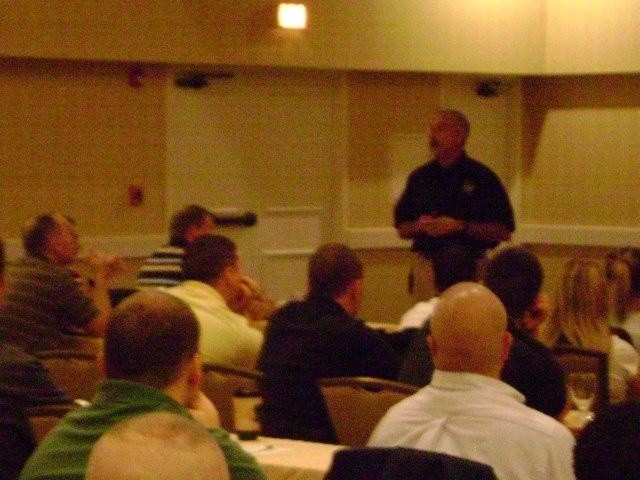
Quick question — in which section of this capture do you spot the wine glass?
[566,374,602,413]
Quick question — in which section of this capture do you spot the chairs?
[1,322,640,478]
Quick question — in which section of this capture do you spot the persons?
[0,205,638,450]
[0,240,75,478]
[366,280,579,479]
[394,112,515,294]
[81,408,231,479]
[571,364,639,480]
[18,289,266,479]
